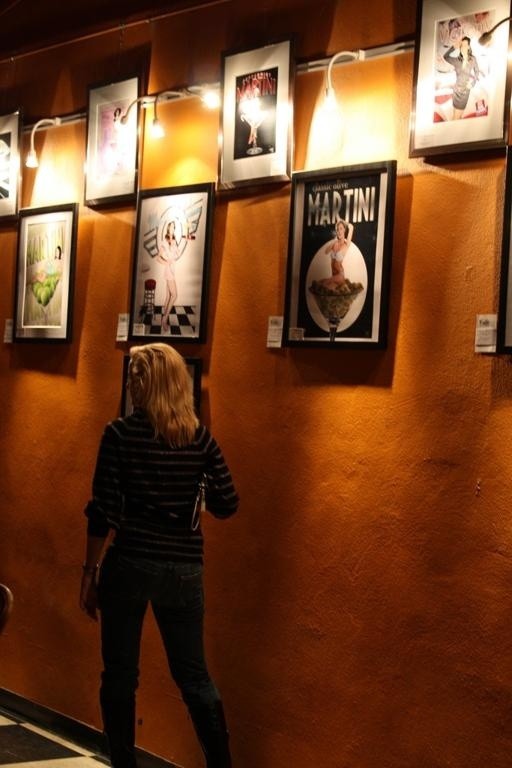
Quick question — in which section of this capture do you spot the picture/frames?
[127,181,216,344]
[0,104,21,229]
[405,0,512,160]
[81,67,144,208]
[213,29,300,198]
[494,145,512,356]
[12,202,78,344]
[281,160,399,349]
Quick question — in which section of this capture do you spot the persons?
[443,36,479,121]
[110,107,123,140]
[245,79,259,148]
[157,221,189,330]
[324,220,355,293]
[48,244,63,278]
[78,341,241,766]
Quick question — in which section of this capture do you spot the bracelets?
[82,561,100,572]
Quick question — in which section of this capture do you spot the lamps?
[316,44,366,106]
[23,115,61,168]
[114,80,220,139]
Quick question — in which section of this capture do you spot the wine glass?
[305,286,363,342]
